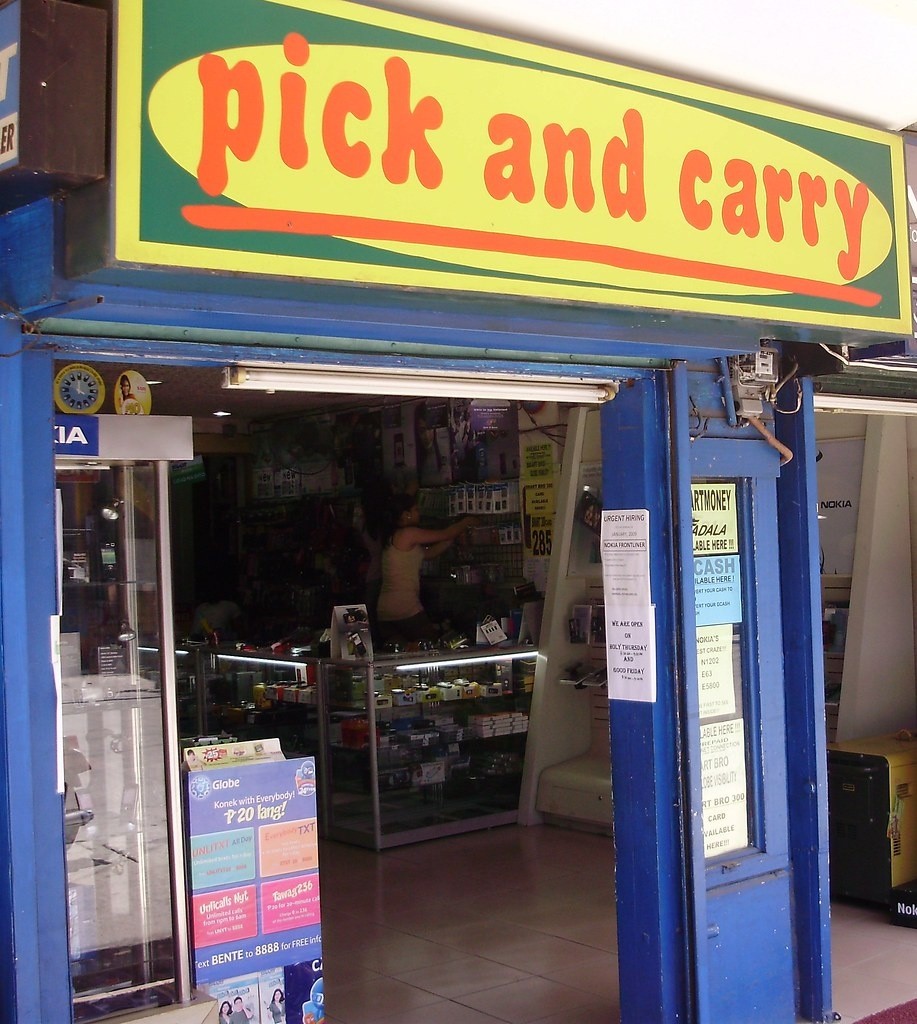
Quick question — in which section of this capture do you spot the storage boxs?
[327,666,529,793]
[230,672,316,703]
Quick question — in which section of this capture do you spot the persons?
[119,375,135,406]
[374,494,480,642]
[219,996,253,1024]
[191,585,243,641]
[186,750,207,766]
[264,989,286,1024]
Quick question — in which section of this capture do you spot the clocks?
[52,362,105,414]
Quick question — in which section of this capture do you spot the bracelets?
[267,1007,270,1010]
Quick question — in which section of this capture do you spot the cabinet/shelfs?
[200,641,330,842]
[51,414,220,1024]
[321,644,540,851]
[135,636,204,739]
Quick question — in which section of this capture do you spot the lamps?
[219,366,620,405]
[116,619,135,641]
[101,497,120,521]
